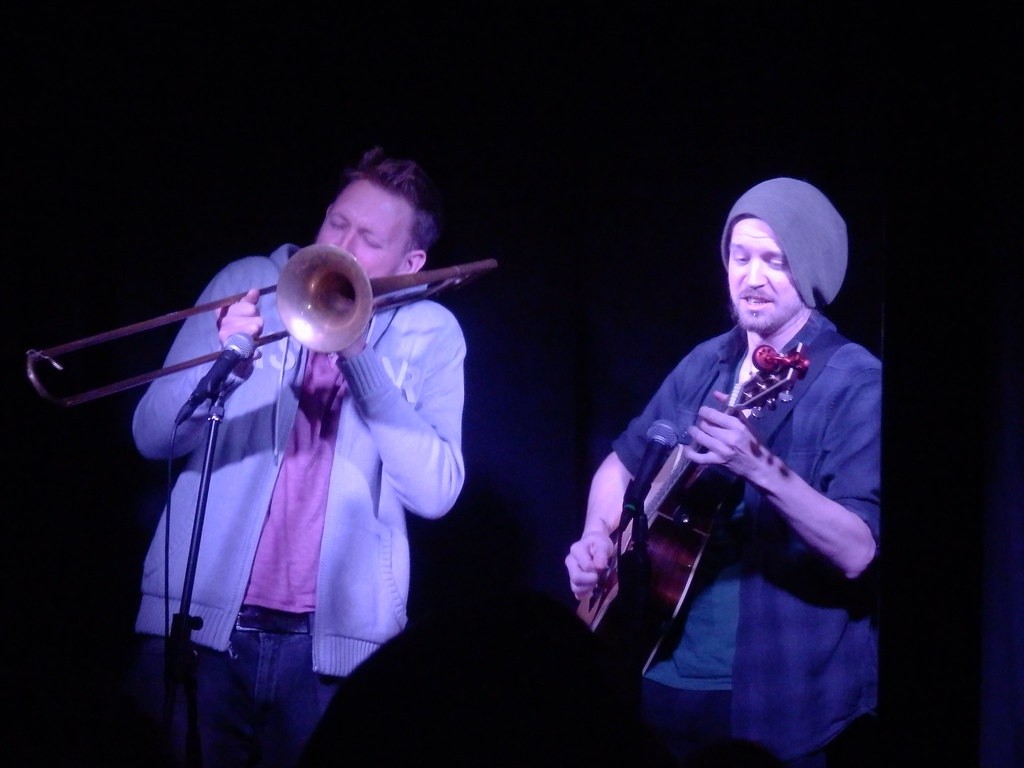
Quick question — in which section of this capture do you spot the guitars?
[575,342,812,677]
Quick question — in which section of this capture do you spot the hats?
[721,177,850,308]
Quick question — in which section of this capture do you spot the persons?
[565,176,884,768]
[128,153,467,768]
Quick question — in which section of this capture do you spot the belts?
[234,604,311,634]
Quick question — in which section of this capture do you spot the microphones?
[173,331,257,424]
[620,420,679,532]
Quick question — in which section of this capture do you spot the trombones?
[26,242,500,412]
